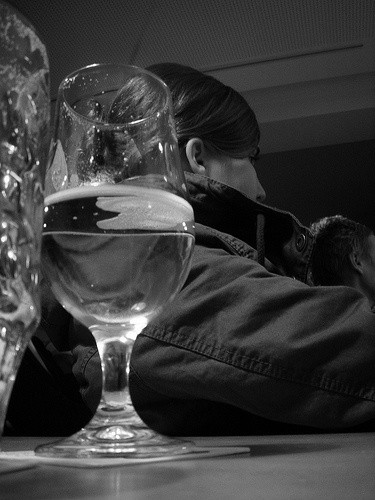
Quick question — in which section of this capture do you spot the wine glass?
[33,62,195,459]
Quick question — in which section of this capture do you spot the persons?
[0,63,375,436]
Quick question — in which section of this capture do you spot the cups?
[0,2,51,435]
